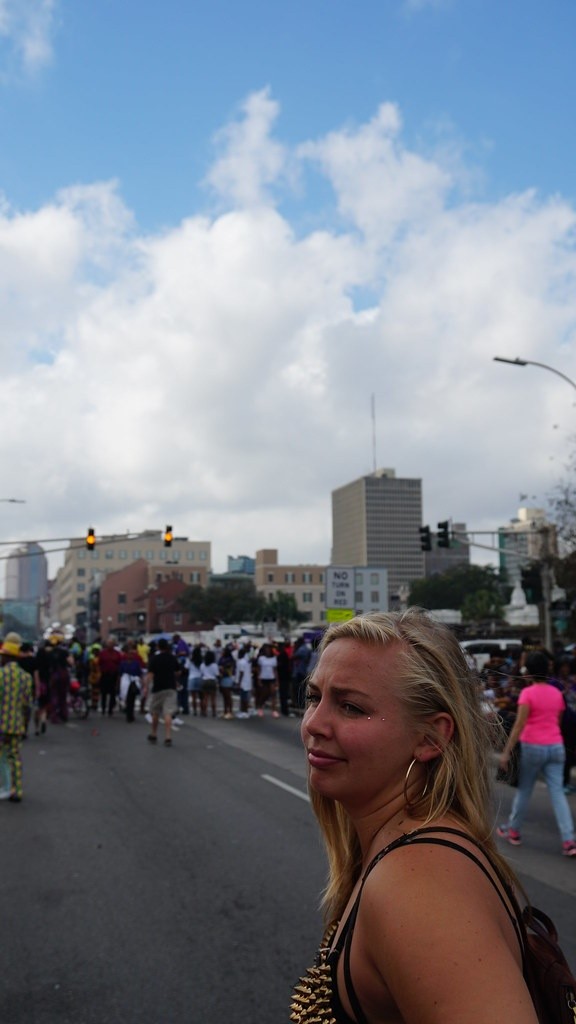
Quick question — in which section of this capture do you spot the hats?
[1,641,20,658]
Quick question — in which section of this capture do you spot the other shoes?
[147,734,157,744]
[235,711,249,720]
[172,718,183,730]
[250,709,256,716]
[272,710,280,718]
[218,711,231,721]
[258,711,262,714]
[144,713,164,724]
[164,739,173,747]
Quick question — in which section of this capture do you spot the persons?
[288,607,539,1024]
[0,624,576,857]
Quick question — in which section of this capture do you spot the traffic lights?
[86,527,95,550]
[164,526,174,546]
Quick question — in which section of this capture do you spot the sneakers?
[560,840,576,856]
[497,826,522,846]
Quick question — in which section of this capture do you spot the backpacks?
[521,899,576,1024]
